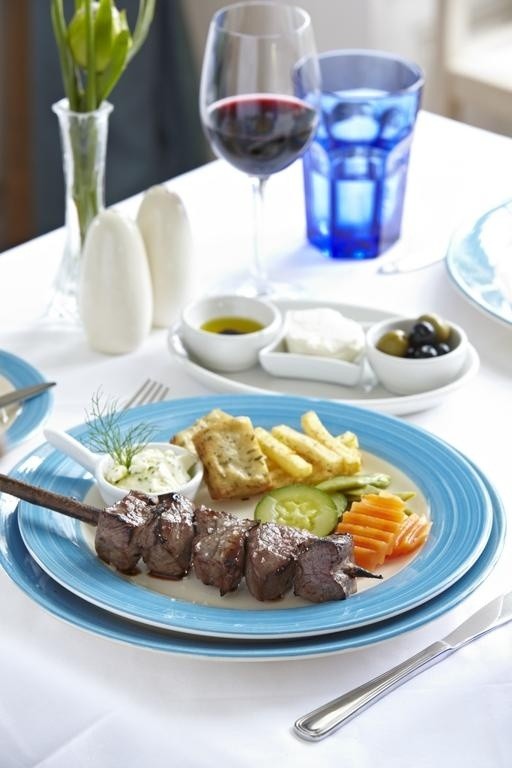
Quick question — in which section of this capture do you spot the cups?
[168,292,282,374]
[289,48,424,261]
[365,313,471,395]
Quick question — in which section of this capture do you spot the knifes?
[288,590,511,744]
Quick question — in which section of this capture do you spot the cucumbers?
[254,482,336,537]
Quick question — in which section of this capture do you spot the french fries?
[255,409,361,480]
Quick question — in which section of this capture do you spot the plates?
[0,394,507,663]
[0,349,53,456]
[444,193,512,330]
[172,304,479,417]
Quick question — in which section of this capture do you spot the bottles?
[76,185,204,355]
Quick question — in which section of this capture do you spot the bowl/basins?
[41,424,205,509]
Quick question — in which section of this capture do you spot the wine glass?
[198,3,322,304]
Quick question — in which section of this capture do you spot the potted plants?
[53,0,158,293]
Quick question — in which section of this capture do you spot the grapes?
[405,322,448,356]
